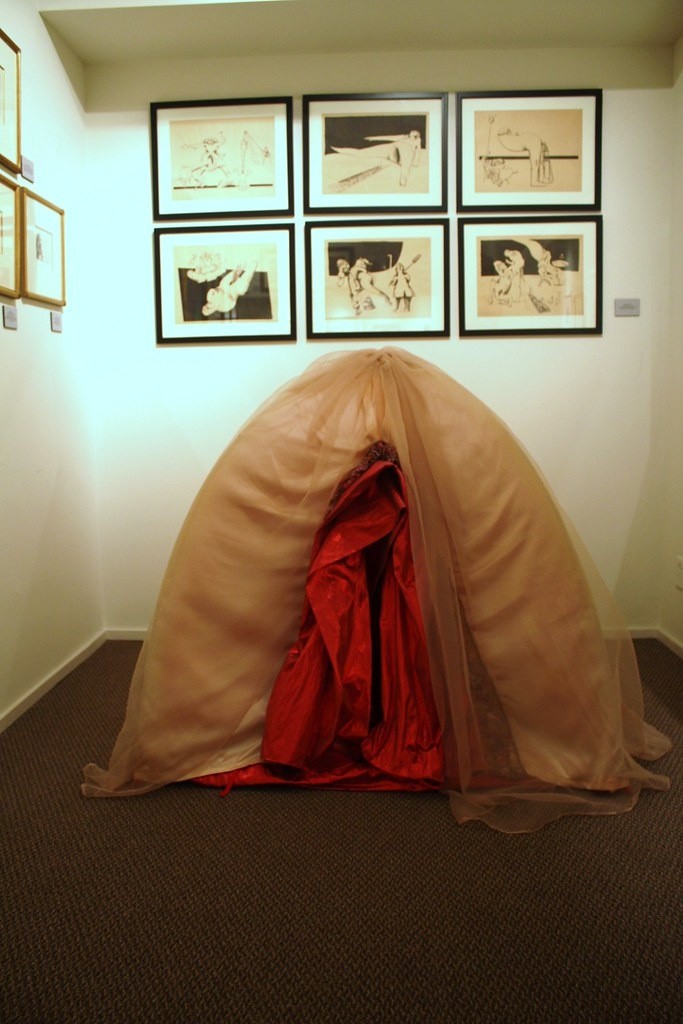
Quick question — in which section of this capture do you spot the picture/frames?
[305,221,450,339]
[150,97,295,220]
[304,94,449,212]
[457,217,603,336]
[0,175,20,300]
[21,185,66,306]
[456,90,602,211]
[153,224,296,344]
[0,27,23,173]
